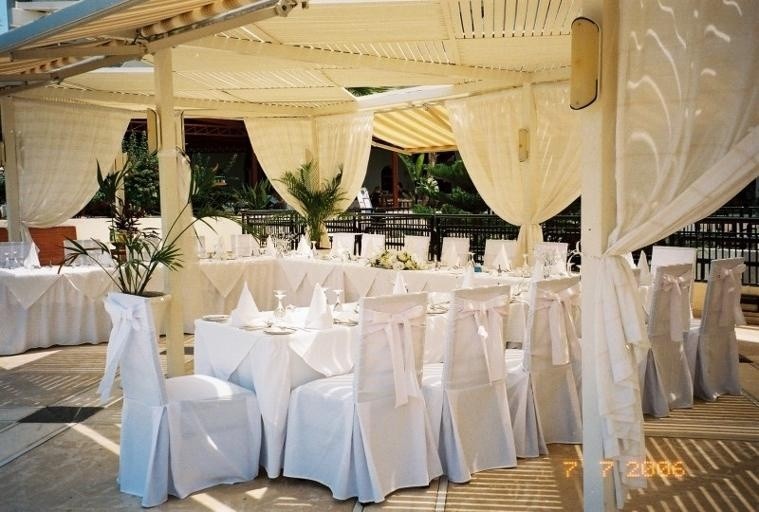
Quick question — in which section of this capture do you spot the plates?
[243,321,270,330]
[266,327,294,334]
[423,305,449,314]
[203,313,230,322]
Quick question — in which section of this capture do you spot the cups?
[322,288,328,305]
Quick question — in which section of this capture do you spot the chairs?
[1,233,582,364]
[421,283,517,485]
[504,275,582,459]
[650,245,697,281]
[641,262,695,418]
[687,256,744,403]
[95,292,263,507]
[283,290,444,505]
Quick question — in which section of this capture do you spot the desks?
[191,292,530,479]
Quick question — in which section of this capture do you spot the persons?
[369,186,387,208]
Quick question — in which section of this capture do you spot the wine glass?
[332,289,345,316]
[460,251,531,278]
[3,251,21,270]
[268,237,359,264]
[273,289,288,317]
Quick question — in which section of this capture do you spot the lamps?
[569,16,602,110]
[176,110,186,154]
[518,128,528,162]
[147,107,161,156]
